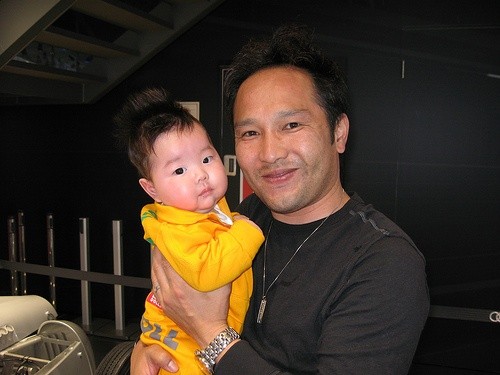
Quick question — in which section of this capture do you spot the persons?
[129,22,431,375]
[112,89,266,375]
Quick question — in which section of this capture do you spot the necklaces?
[256,188,345,324]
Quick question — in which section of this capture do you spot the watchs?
[194,327,241,375]
